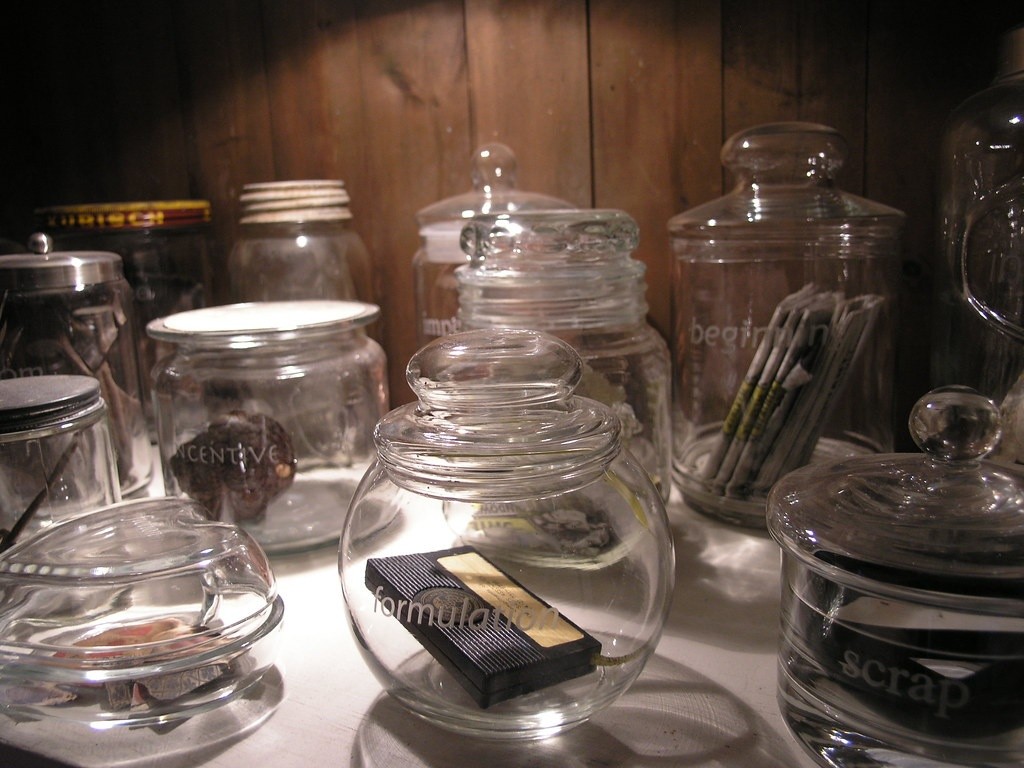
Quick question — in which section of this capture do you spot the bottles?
[0,26,1024,767]
